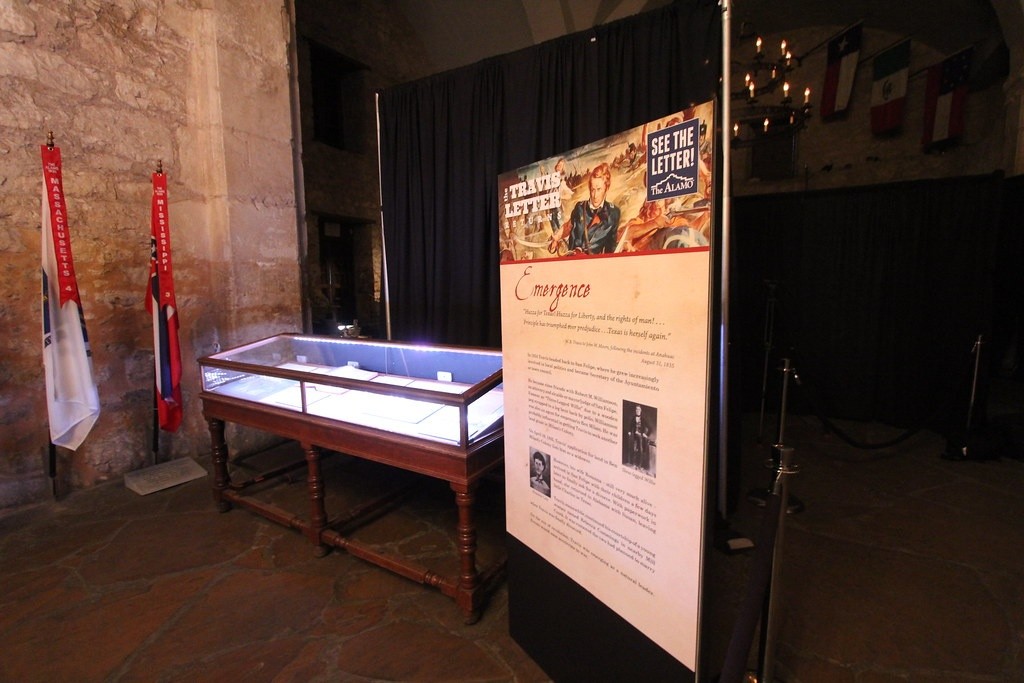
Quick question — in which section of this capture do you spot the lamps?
[719,13,813,148]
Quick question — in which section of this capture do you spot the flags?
[39,143,102,451]
[145,172,183,431]
